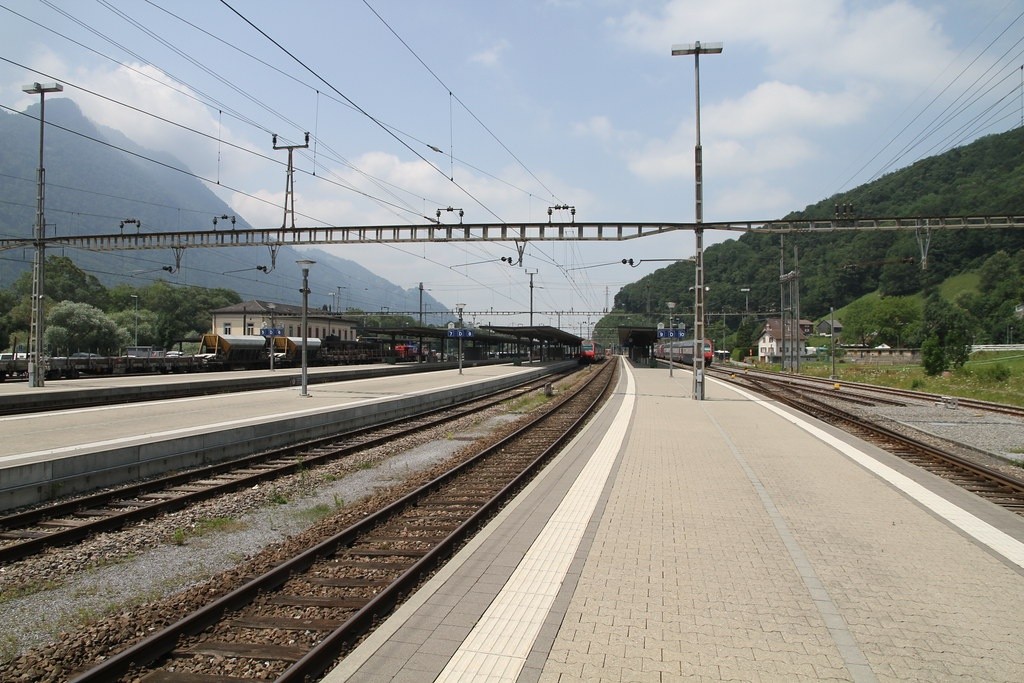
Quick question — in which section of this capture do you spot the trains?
[0,335,437,384]
[648,339,713,366]
[580,340,605,363]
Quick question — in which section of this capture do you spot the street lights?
[667,302,676,376]
[131,294,138,357]
[296,260,317,396]
[456,303,467,375]
[22,82,62,387]
[266,302,276,369]
[671,41,725,399]
